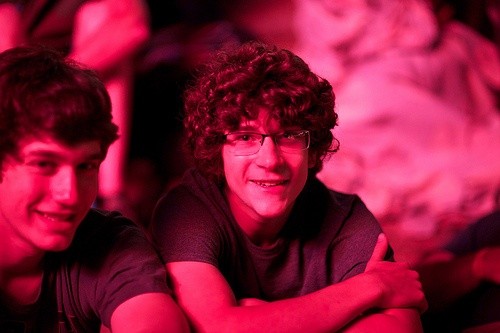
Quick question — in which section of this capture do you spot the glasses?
[216,129,310,156]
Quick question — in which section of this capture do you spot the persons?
[411,205,500,333]
[0,0,154,246]
[1,45,191,332]
[150,37,429,331]
